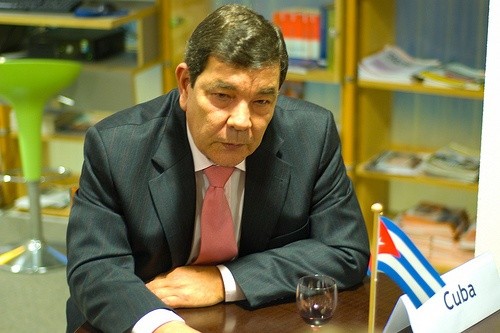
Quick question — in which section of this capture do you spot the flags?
[367,217,445,308]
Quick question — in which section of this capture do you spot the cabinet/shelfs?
[0,1,163,215]
[165,0,492,274]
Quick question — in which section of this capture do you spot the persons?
[65,5,370,333]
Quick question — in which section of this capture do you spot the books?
[364,152,479,181]
[359,47,485,90]
[391,201,476,252]
[272,9,335,69]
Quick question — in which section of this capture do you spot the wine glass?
[297,273,337,333]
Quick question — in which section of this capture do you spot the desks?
[72,271,500,333]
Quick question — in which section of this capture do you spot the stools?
[0,60,83,274]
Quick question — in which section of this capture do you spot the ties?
[193,166,237,266]
